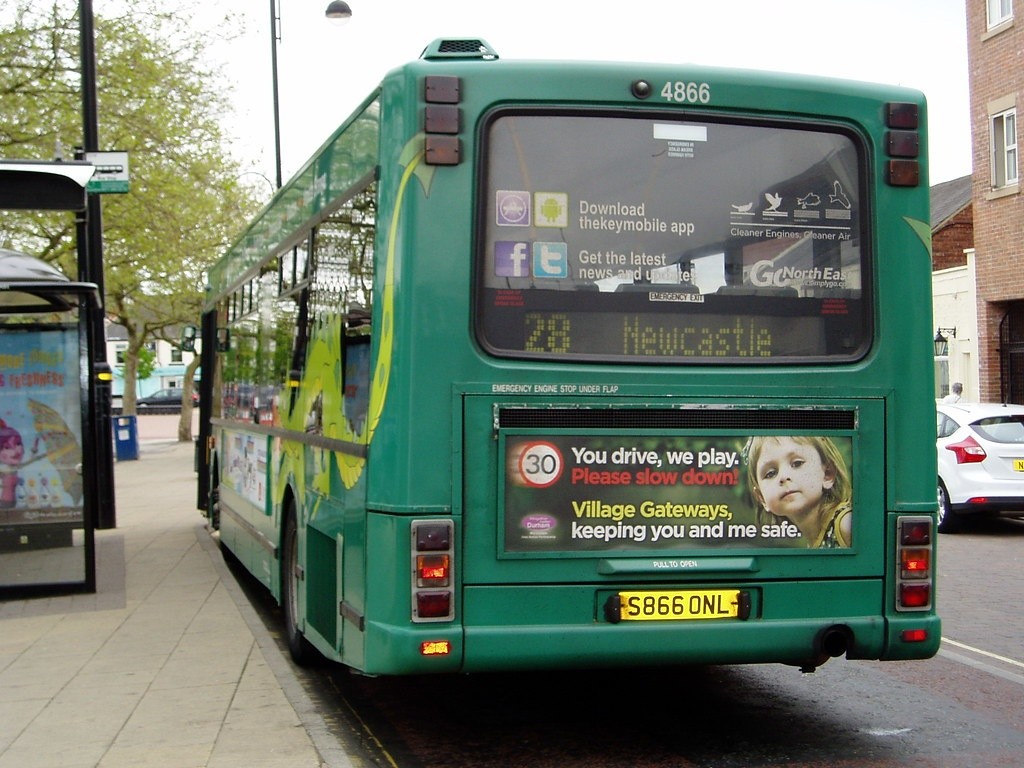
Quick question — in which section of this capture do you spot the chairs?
[615,284,700,293]
[813,288,861,300]
[716,286,799,297]
[487,280,600,292]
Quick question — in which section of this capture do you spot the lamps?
[934,326,956,356]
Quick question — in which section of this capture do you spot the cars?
[934,400,1024,534]
[136,387,201,416]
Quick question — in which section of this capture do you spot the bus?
[180,42,940,681]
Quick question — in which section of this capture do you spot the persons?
[938,382,962,437]
[747,436,852,549]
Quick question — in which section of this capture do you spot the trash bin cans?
[112,414,139,461]
